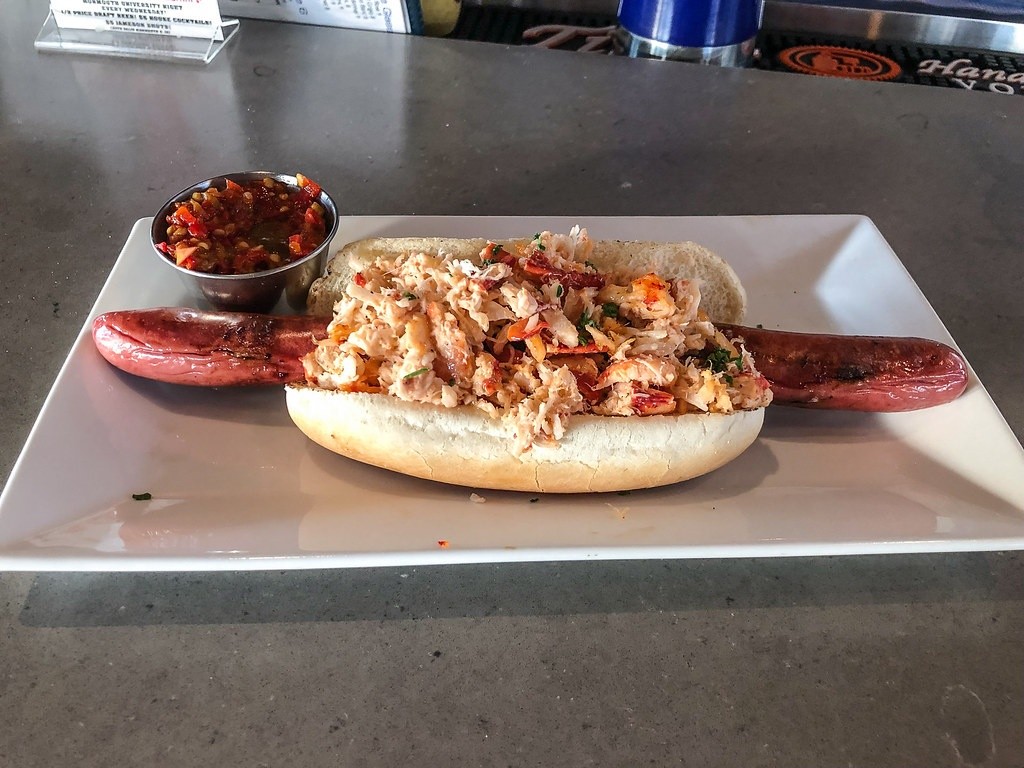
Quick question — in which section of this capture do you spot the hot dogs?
[86,225,968,496]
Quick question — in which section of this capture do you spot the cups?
[611,0,765,68]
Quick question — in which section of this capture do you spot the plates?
[0,215,1024,574]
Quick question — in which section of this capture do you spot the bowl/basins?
[152,171,339,316]
